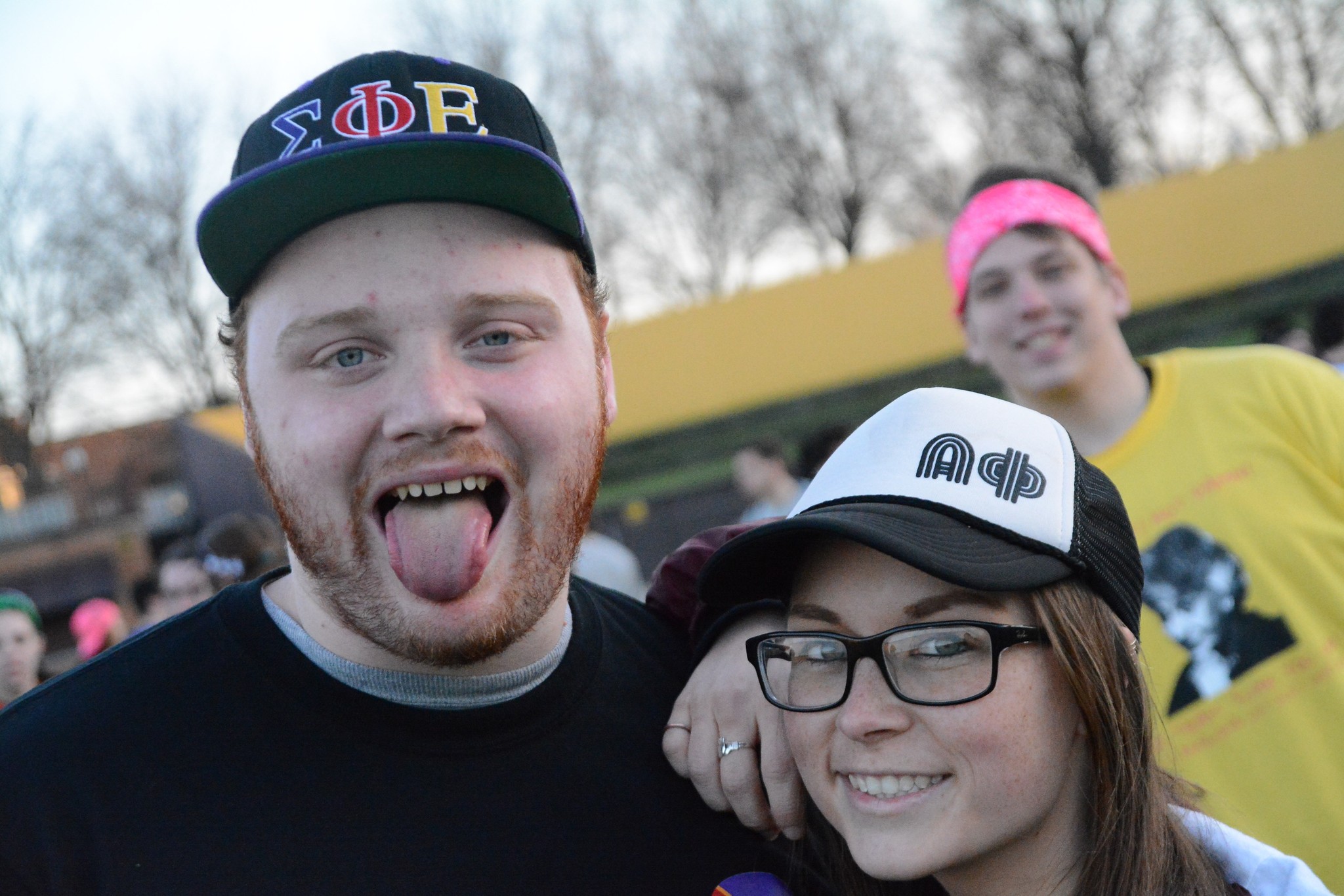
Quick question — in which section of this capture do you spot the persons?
[0,508,287,713]
[726,427,814,527]
[710,384,1330,896]
[943,159,1344,894]
[0,49,855,896]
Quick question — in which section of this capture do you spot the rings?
[718,737,758,757]
[665,722,692,735]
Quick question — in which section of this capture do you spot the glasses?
[746,618,1054,715]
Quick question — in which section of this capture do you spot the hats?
[693,387,1141,653]
[71,597,118,661]
[0,587,47,638]
[195,51,597,311]
